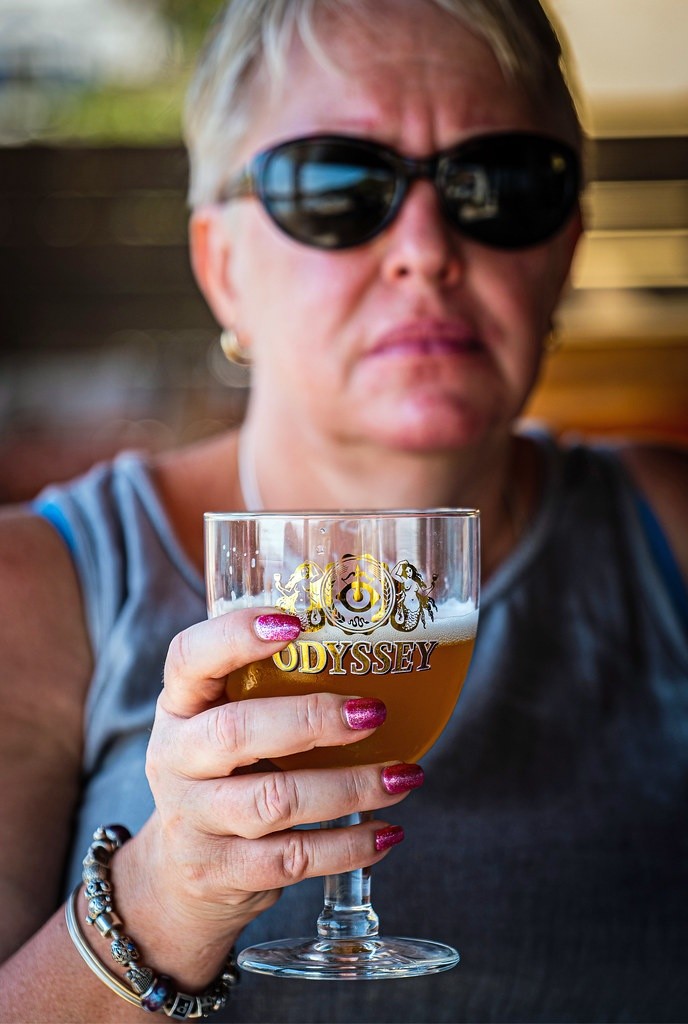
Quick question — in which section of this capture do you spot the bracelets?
[82,821,242,1017]
[61,884,151,1015]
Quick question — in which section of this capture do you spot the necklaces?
[235,424,522,611]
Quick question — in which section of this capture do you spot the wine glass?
[202,505,475,982]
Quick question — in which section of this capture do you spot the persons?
[0,0,688,1022]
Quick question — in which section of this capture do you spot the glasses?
[205,128,587,252]
